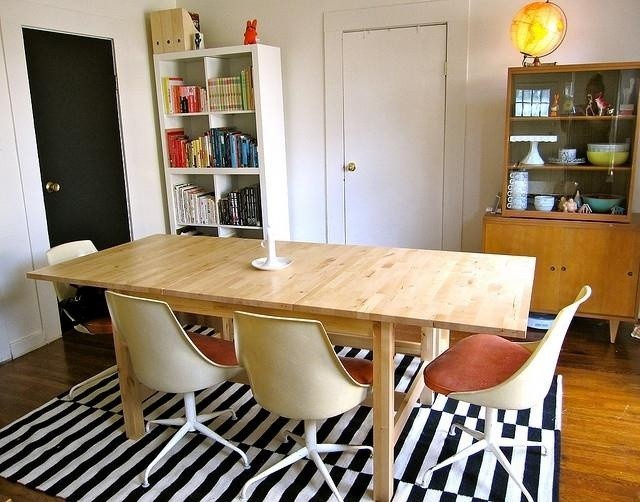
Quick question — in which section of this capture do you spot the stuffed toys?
[244,19,257,45]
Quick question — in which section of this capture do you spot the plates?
[548,158,585,165]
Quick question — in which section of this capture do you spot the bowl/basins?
[534,196,555,211]
[587,151,629,166]
[587,143,630,151]
[582,193,625,212]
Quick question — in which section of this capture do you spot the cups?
[557,149,577,160]
[507,171,529,210]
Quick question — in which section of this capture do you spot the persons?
[194,33,201,50]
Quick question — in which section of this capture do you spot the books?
[218,182,262,227]
[166,126,259,169]
[208,66,255,112]
[173,182,218,225]
[162,76,207,114]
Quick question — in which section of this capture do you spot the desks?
[27,234,538,440]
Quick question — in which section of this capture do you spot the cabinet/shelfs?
[484,211,640,344]
[150,42,290,239]
[500,59,638,222]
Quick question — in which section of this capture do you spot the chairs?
[232,311,373,502]
[46,238,113,338]
[420,285,593,501]
[105,289,251,489]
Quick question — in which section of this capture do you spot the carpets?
[2,321,565,501]
